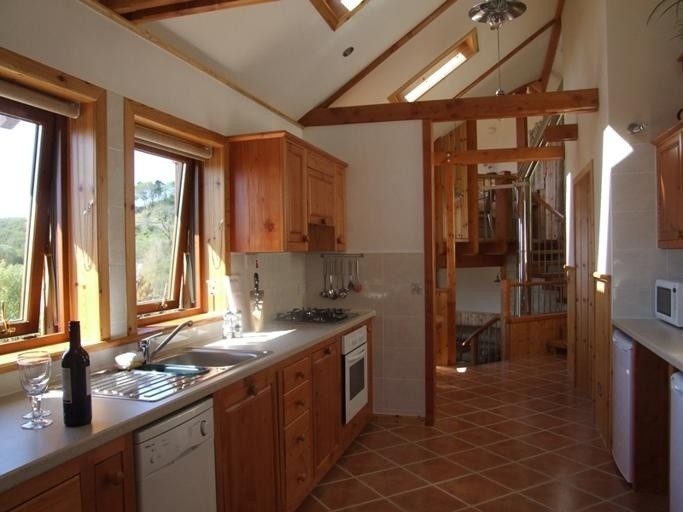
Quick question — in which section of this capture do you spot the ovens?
[342,325,369,425]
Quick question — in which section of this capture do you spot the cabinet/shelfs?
[310,143,335,227]
[230,131,309,256]
[309,155,350,252]
[343,405,370,448]
[0,432,136,511]
[277,349,317,511]
[611,313,683,509]
[313,333,343,488]
[592,273,610,450]
[214,362,277,511]
[650,123,683,247]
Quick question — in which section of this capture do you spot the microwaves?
[654,279,683,327]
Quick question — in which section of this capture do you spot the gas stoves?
[274,306,360,326]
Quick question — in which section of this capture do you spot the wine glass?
[19,359,52,429]
[18,351,50,418]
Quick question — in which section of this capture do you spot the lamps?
[468,1,527,95]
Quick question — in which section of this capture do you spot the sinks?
[88,363,209,380]
[156,348,258,368]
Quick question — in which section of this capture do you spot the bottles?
[232,307,242,336]
[222,309,233,338]
[61,321,92,427]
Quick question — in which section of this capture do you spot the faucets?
[140,321,195,360]
